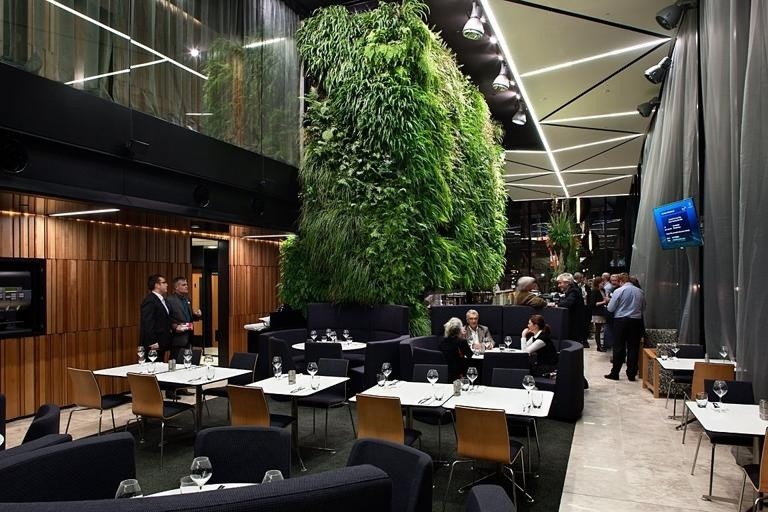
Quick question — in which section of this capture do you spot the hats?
[518,277,535,291]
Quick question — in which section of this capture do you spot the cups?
[273,367,282,380]
[474,348,480,356]
[661,349,669,361]
[310,376,322,391]
[179,476,202,493]
[434,387,446,402]
[262,469,285,485]
[460,378,469,390]
[529,392,545,411]
[695,391,708,409]
[375,372,386,387]
[347,337,353,345]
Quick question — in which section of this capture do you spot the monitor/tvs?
[652,196,704,250]
[0,257,46,339]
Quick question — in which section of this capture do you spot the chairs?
[20,402,62,444]
[196,426,293,484]
[413,363,457,450]
[352,342,400,386]
[346,437,435,510]
[356,395,435,490]
[492,368,542,473]
[638,329,768,512]
[482,351,529,387]
[64,367,146,447]
[198,352,259,421]
[174,349,212,418]
[303,342,343,373]
[268,335,297,377]
[297,359,357,446]
[441,406,527,510]
[127,373,197,471]
[467,484,519,510]
[226,384,296,465]
[413,347,445,367]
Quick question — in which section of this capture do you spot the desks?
[291,341,368,352]
[350,379,457,454]
[246,371,350,473]
[155,363,253,437]
[93,361,189,381]
[476,346,522,358]
[441,384,555,418]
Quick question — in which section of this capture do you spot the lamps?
[637,97,661,117]
[492,62,510,92]
[462,3,484,41]
[655,0,696,30]
[644,56,671,84]
[512,99,526,125]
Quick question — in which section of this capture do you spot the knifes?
[289,386,305,393]
[388,380,399,386]
[417,396,431,404]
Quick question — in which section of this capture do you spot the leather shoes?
[626,369,635,381]
[605,374,619,380]
[597,348,607,352]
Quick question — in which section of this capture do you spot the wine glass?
[428,368,438,394]
[466,367,478,392]
[272,356,283,382]
[381,362,391,382]
[307,362,319,380]
[670,341,680,361]
[308,327,349,345]
[711,379,729,409]
[719,345,729,363]
[482,335,513,351]
[522,375,538,401]
[114,478,143,499]
[183,348,215,380]
[189,456,214,491]
[136,345,159,373]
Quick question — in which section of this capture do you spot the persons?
[165,276,203,399]
[462,309,496,352]
[548,267,646,390]
[443,317,473,360]
[515,275,546,308]
[521,315,557,376]
[140,272,171,363]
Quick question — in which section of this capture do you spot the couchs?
[401,302,583,425]
[256,302,411,393]
[4,431,393,510]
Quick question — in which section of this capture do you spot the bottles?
[453,379,461,395]
[759,399,768,419]
[288,369,296,384]
[168,359,176,370]
